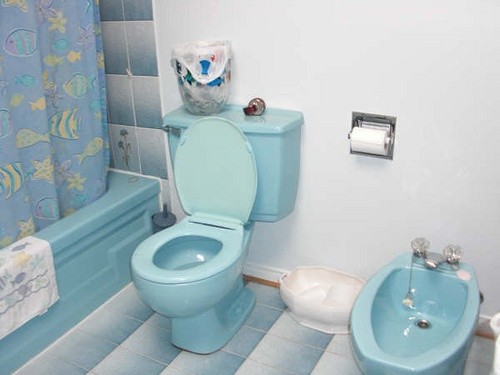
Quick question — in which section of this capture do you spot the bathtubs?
[0,170,155,269]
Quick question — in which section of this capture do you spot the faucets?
[425,252,445,269]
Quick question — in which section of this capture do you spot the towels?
[0,236,61,341]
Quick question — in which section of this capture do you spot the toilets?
[129,103,303,355]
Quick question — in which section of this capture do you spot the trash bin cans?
[169,39,232,115]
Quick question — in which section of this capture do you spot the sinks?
[350,253,479,371]
